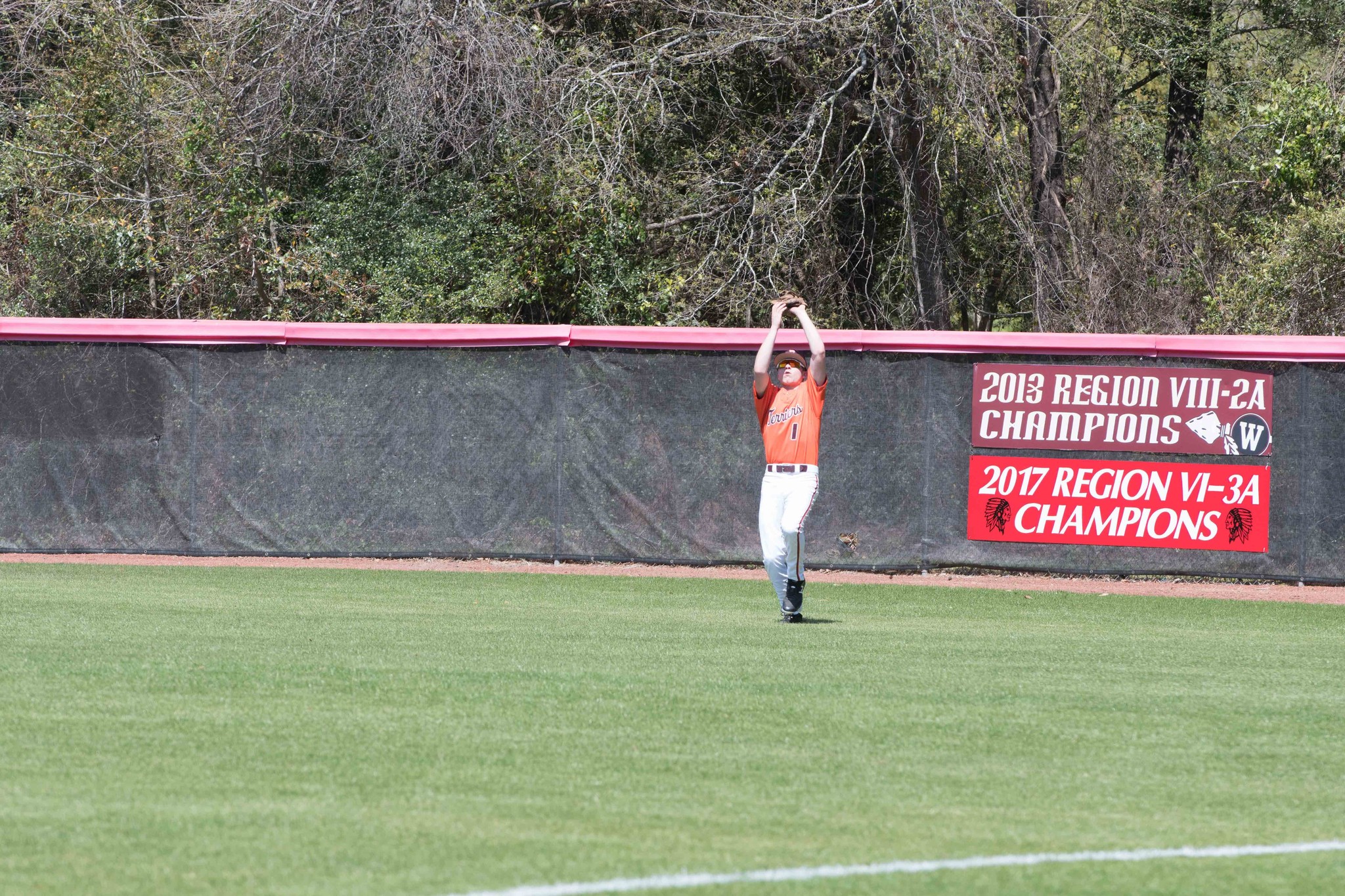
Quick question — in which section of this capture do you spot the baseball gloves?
[769,289,808,318]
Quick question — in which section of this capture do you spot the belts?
[768,465,808,473]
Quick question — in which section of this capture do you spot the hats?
[774,349,808,369]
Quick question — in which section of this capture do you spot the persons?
[753,297,829,623]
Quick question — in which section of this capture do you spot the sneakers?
[778,613,803,623]
[781,578,806,615]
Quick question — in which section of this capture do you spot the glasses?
[778,360,802,368]
